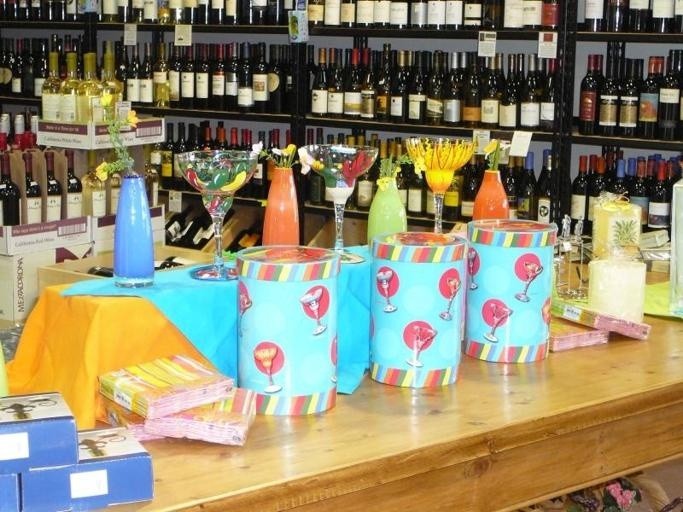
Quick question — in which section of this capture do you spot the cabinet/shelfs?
[92,0,296,260]
[564,0,683,236]
[296,0,562,246]
[0,1,92,108]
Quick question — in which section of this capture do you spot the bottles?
[103,40,121,125]
[573,145,681,237]
[1,0,557,27]
[1,154,22,226]
[2,112,14,172]
[45,152,62,222]
[83,149,106,217]
[64,150,82,218]
[577,49,681,141]
[553,212,585,300]
[40,52,60,122]
[262,168,302,248]
[305,40,555,128]
[79,53,101,119]
[13,113,26,171]
[366,177,408,247]
[108,148,120,218]
[113,175,153,287]
[306,131,552,215]
[142,145,159,209]
[23,152,42,222]
[61,54,77,124]
[28,115,40,174]
[473,169,510,220]
[1,36,291,118]
[151,119,291,187]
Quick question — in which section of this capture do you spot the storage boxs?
[0,238,93,331]
[36,244,217,298]
[1,119,219,331]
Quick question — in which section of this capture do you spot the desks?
[83,233,682,511]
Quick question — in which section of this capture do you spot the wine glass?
[540,297,551,342]
[515,263,544,303]
[300,288,327,335]
[406,326,437,367]
[253,347,282,396]
[437,276,461,321]
[236,287,252,335]
[465,250,477,291]
[482,303,514,344]
[375,268,396,313]
[329,338,338,383]
